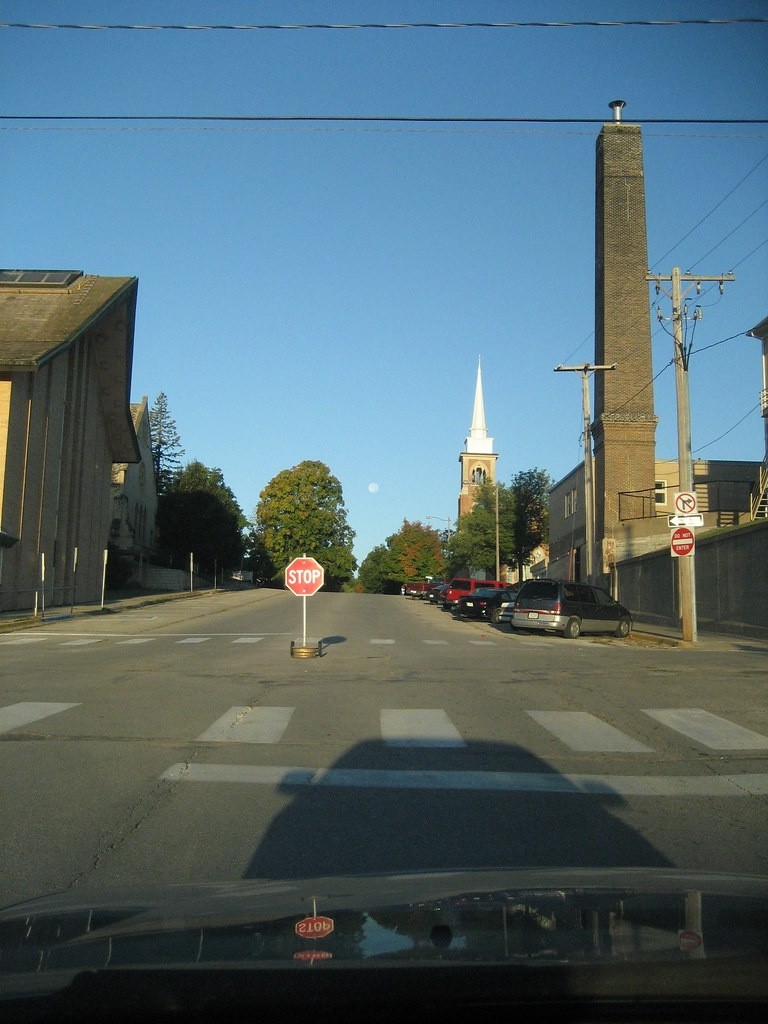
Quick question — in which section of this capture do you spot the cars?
[456,587,517,624]
[424,583,448,604]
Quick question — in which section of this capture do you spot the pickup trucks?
[405,580,445,599]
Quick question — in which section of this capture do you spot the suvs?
[511,578,634,641]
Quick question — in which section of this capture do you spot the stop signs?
[285,557,325,598]
[670,527,695,554]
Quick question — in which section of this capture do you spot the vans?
[443,577,513,611]
